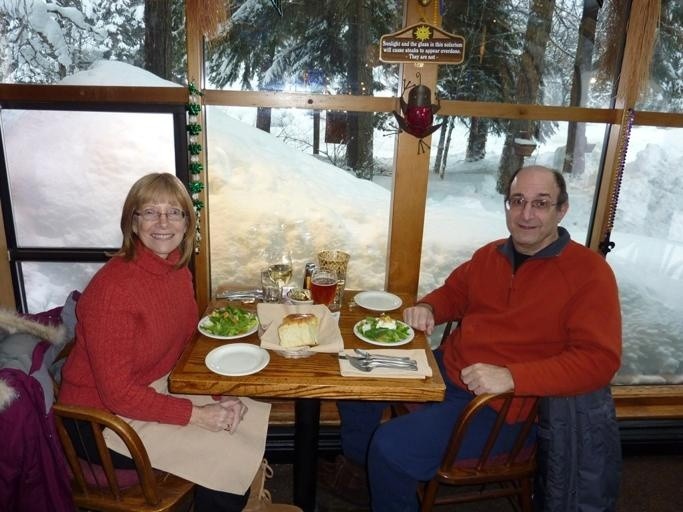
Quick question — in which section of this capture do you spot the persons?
[336,167,621,510]
[58,172,250,512]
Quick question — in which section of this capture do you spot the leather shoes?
[319,460,370,505]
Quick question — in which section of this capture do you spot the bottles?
[303,262,317,289]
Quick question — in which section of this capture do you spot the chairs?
[388,319,542,512]
[49,401,196,511]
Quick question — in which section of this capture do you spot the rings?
[222,424,230,431]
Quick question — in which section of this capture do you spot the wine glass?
[266,249,293,300]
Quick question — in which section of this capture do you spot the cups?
[317,250,351,310]
[259,268,280,305]
[309,265,337,306]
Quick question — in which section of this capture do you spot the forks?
[346,356,420,372]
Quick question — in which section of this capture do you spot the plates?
[352,318,416,347]
[285,289,313,305]
[197,313,263,340]
[353,290,403,312]
[203,342,270,379]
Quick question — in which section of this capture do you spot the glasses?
[510,196,557,210]
[133,208,186,220]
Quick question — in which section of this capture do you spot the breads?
[277,313,319,349]
